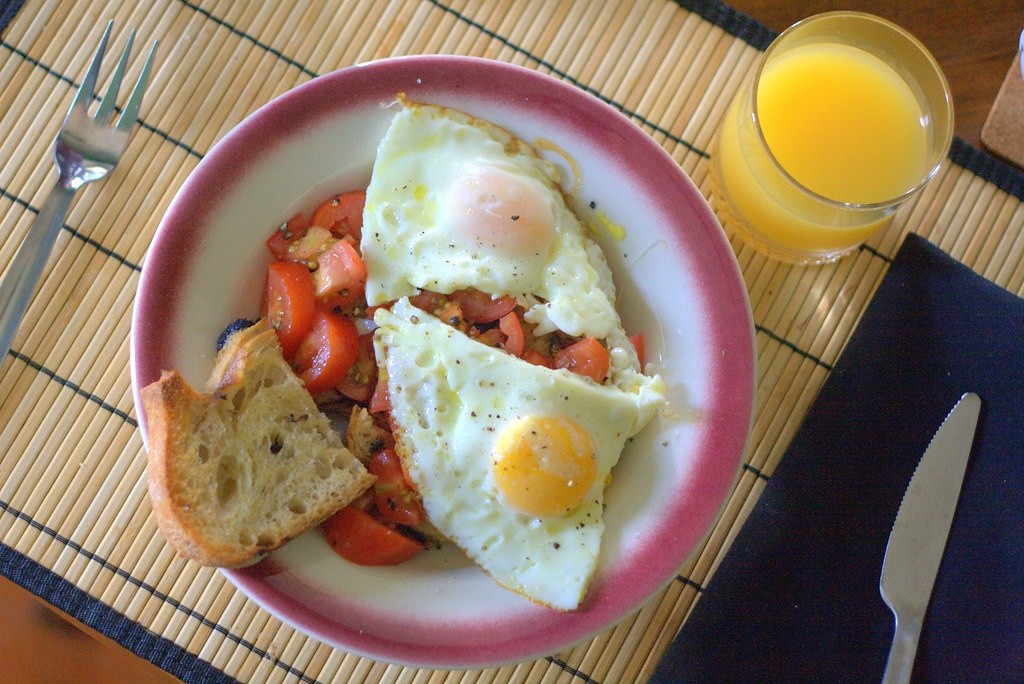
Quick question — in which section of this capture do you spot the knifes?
[879,392,982,684]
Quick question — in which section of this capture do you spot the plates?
[130,54,758,671]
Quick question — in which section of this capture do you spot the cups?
[711,11,954,264]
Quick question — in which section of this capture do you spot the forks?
[0,19,159,367]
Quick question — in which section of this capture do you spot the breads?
[139,315,378,566]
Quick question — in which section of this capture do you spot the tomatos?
[267,190,644,564]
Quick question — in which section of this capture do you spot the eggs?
[372,295,664,612]
[359,94,623,342]
